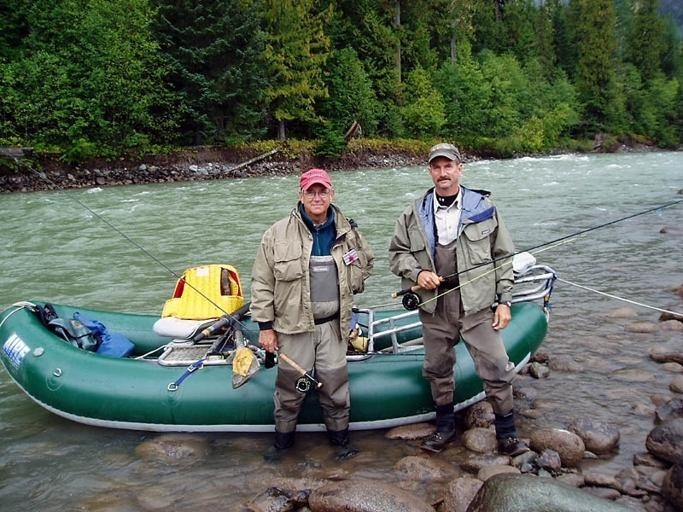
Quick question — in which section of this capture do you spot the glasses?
[299,188,334,200]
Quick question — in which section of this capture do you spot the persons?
[386,142,532,457]
[248,169,376,465]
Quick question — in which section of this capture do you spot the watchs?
[499,300,512,307]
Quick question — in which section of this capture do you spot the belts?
[313,309,341,325]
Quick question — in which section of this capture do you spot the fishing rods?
[390,198,683,311]
[0,147,323,389]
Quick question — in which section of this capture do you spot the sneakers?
[261,440,296,460]
[418,427,458,453]
[497,436,531,457]
[328,440,359,460]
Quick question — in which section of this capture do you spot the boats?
[0,299,547,433]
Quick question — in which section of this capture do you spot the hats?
[426,143,462,167]
[298,167,332,190]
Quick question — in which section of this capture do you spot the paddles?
[231,321,260,387]
[192,302,251,345]
